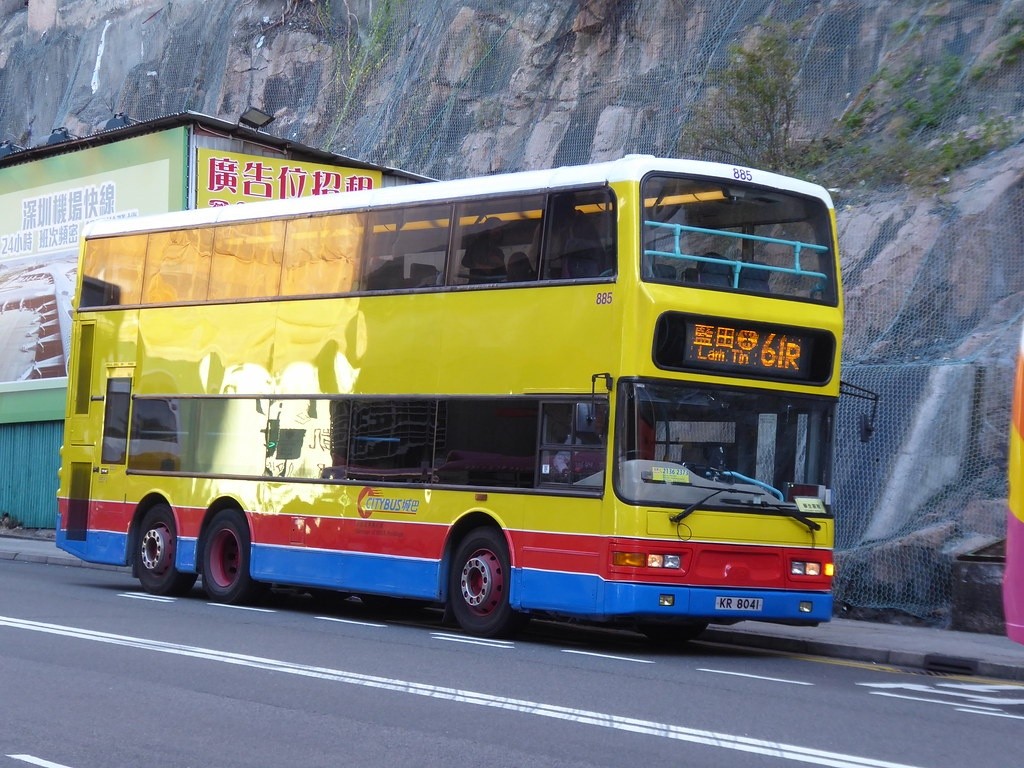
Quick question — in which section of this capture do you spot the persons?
[461,192,606,285]
[553,431,592,475]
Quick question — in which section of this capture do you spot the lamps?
[237,106,275,134]
[0,141,28,157]
[103,113,142,131]
[47,127,77,145]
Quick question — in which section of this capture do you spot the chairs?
[409,206,771,294]
[687,447,703,464]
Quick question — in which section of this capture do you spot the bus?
[56,153,883,642]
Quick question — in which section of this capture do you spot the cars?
[106,378,668,503]
